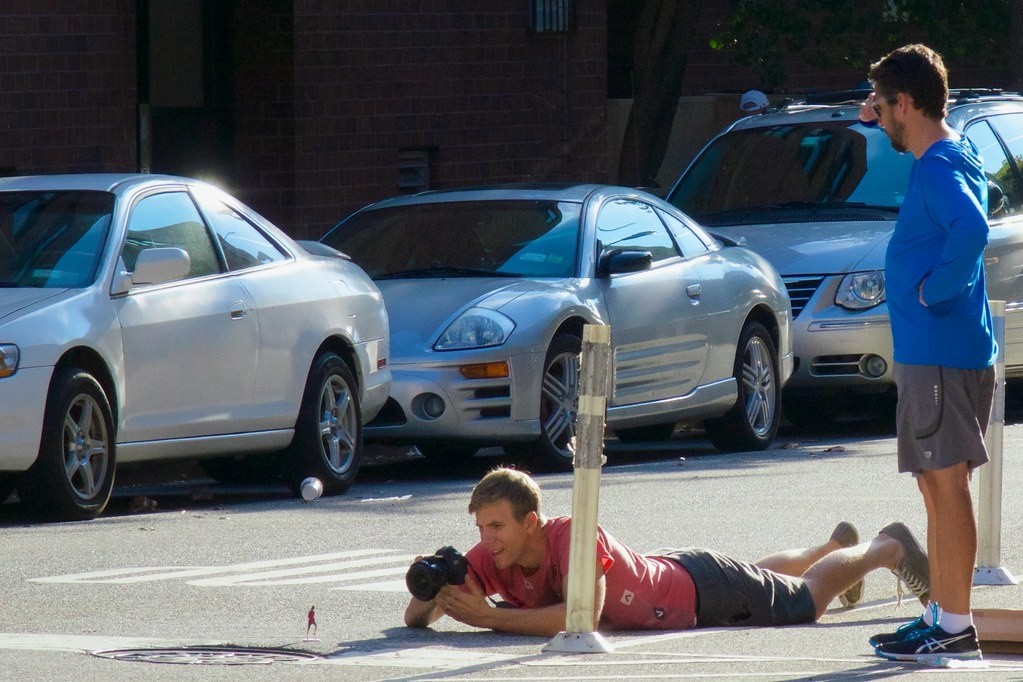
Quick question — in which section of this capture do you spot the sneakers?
[828,521,865,608]
[868,614,932,648]
[875,625,983,668]
[878,521,929,612]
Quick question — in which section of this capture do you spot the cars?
[310,179,793,483]
[1,173,393,522]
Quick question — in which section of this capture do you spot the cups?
[300,477,323,502]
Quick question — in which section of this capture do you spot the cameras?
[406,545,468,602]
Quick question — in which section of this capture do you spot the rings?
[445,604,451,612]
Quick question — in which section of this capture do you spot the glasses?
[871,96,914,118]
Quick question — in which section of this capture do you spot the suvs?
[660,88,1023,432]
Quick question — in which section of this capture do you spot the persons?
[307,605,317,639]
[859,44,999,660]
[740,90,769,115]
[403,468,931,637]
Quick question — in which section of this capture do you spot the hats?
[739,90,769,112]
